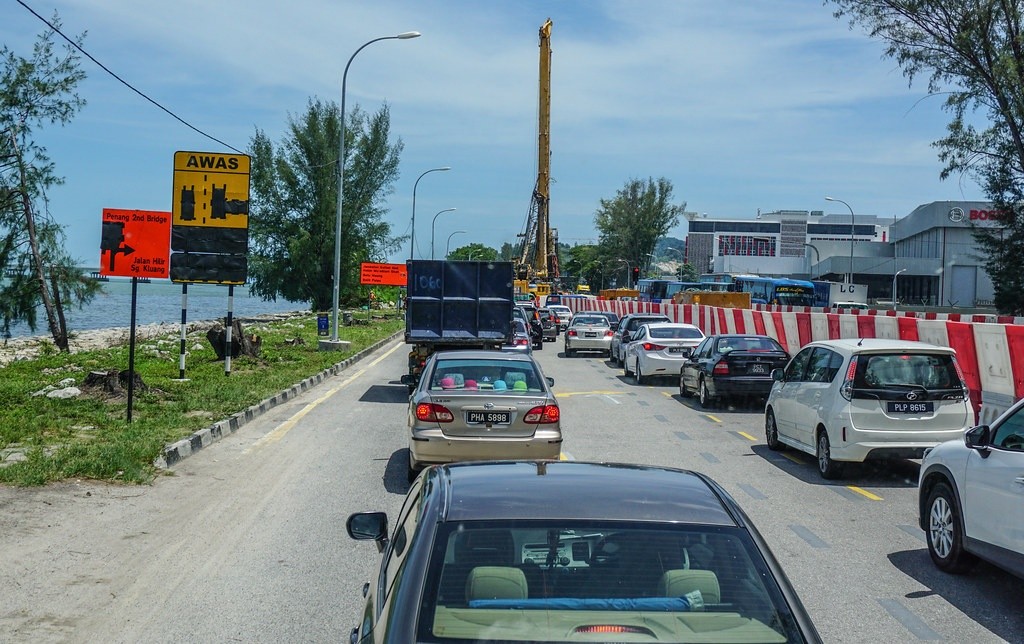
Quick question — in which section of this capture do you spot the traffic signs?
[99,208,173,281]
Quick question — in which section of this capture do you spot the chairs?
[657,569,721,603]
[576,320,602,324]
[440,529,542,604]
[443,373,464,386]
[504,372,527,389]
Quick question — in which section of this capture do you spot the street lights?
[432,208,457,260]
[469,249,482,261]
[410,166,452,260]
[617,259,630,290]
[894,268,907,310]
[825,197,854,285]
[447,230,467,260]
[329,30,423,342]
[713,237,731,274]
[668,246,682,282]
[646,253,659,280]
[573,260,582,279]
[593,260,604,290]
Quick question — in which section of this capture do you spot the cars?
[346,457,823,644]
[493,292,573,355]
[564,309,791,410]
[401,350,563,485]
[917,398,1024,581]
[765,339,975,480]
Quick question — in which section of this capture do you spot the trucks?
[405,259,515,396]
[810,279,870,309]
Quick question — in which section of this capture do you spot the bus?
[636,273,818,308]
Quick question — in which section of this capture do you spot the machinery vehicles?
[509,16,591,297]
